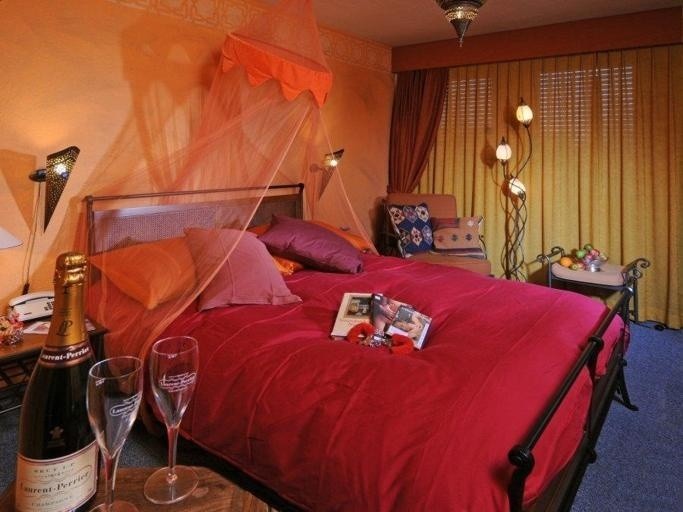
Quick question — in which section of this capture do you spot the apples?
[570,244,600,270]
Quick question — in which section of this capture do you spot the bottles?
[15,251,101,512]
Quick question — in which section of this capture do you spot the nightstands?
[1,314,110,414]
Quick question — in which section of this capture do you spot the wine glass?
[577,255,609,272]
[86,336,199,511]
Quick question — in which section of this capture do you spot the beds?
[86,183,640,511]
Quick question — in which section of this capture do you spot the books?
[330,293,433,351]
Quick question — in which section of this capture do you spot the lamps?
[19,146,80,295]
[494,97,534,281]
[310,150,347,202]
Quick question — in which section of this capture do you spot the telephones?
[9,291,54,322]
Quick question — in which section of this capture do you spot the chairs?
[383,194,492,276]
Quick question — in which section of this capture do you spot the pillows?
[387,204,487,259]
[87,213,370,311]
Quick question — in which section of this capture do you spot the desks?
[543,245,650,325]
